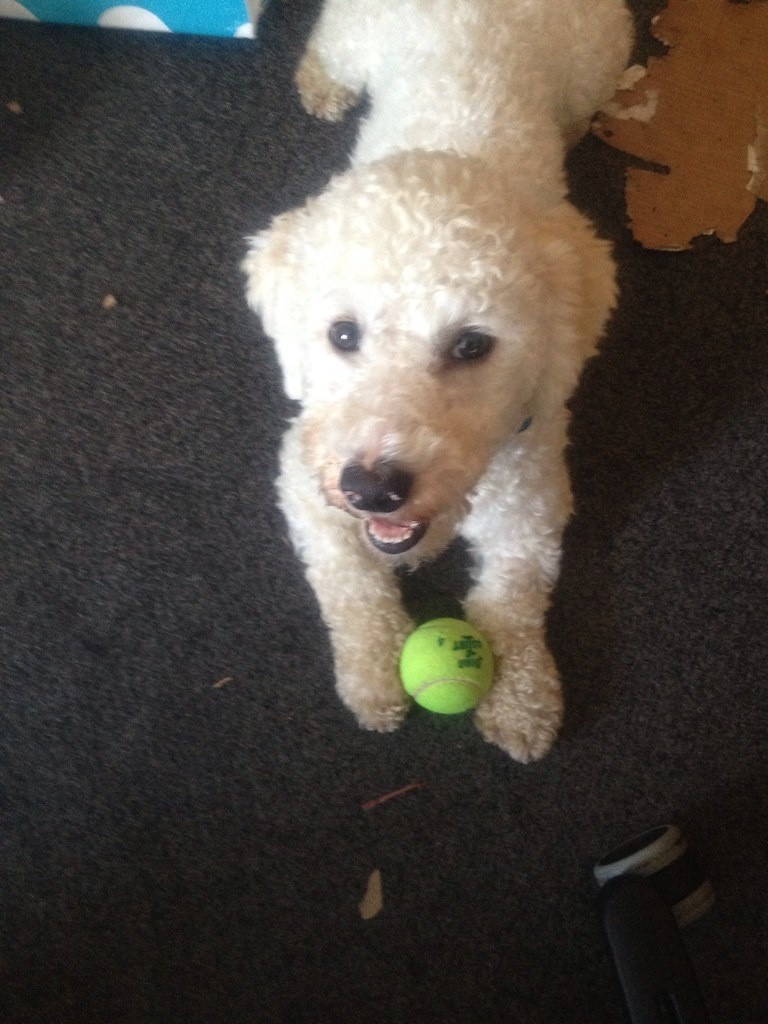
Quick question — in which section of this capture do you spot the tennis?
[399,617,494,716]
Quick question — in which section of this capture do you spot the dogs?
[235,0,636,766]
